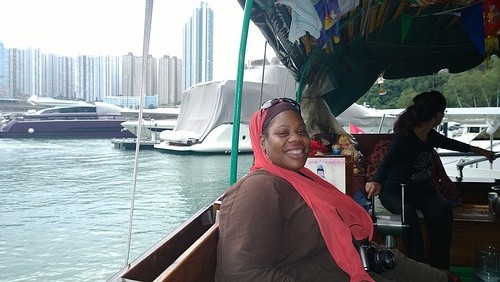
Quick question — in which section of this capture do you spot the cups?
[488,192,497,211]
[479,246,500,279]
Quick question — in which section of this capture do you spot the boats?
[153,65,396,154]
[1,104,144,139]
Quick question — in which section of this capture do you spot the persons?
[215,98,448,282]
[365,90,498,282]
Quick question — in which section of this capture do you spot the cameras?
[359,244,397,276]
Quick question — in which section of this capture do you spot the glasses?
[260,97,301,116]
